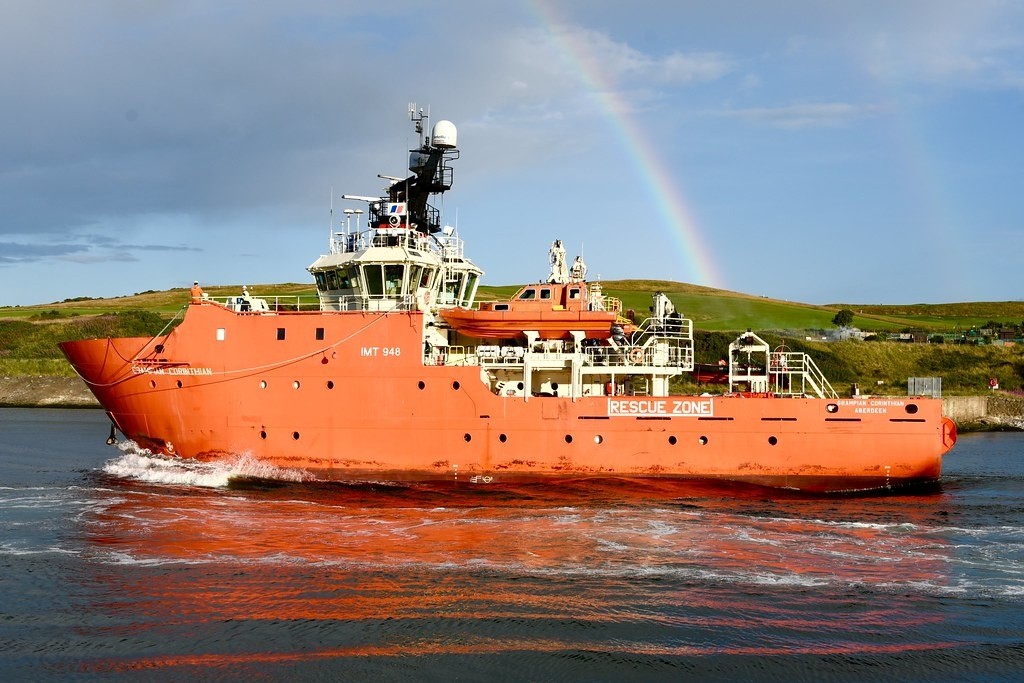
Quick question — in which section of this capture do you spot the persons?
[240,285,251,315]
[190,281,203,304]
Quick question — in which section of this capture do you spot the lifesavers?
[630,348,644,363]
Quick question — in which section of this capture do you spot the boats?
[687,360,777,384]
[55,102,961,499]
[436,280,619,345]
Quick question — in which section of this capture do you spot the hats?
[242,286,248,288]
[194,281,198,283]
